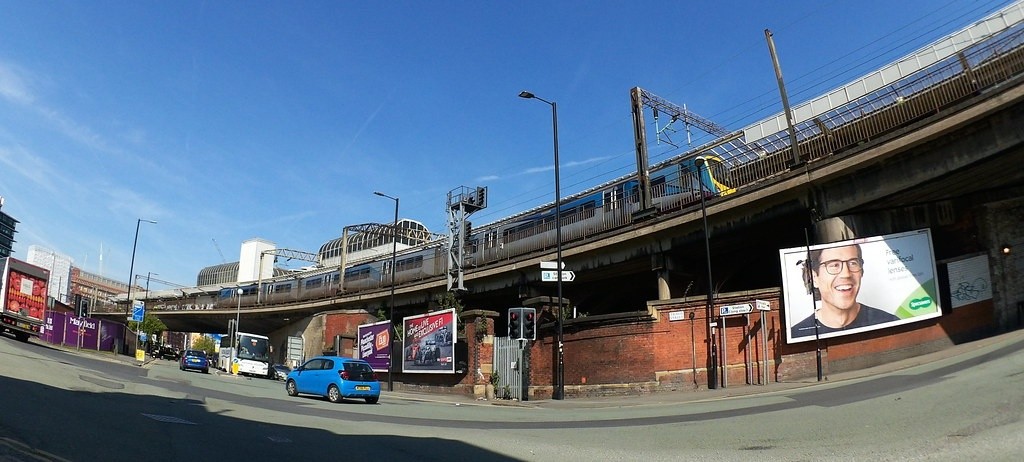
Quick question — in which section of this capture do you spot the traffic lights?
[508,308,522,340]
[522,308,537,340]
[79,300,88,318]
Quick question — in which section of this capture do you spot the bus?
[217,332,273,378]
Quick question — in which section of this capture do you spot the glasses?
[819,257,864,276]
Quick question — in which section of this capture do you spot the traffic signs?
[542,271,576,281]
[720,304,753,315]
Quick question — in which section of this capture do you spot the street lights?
[374,191,399,393]
[143,272,159,346]
[123,218,157,354]
[694,156,718,389]
[518,91,564,400]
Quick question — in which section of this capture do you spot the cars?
[268,364,291,382]
[286,356,381,405]
[151,346,219,374]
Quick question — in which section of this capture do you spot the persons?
[426,346,432,354]
[791,244,901,338]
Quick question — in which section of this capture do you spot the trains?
[213,155,737,310]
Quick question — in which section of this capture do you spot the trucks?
[0,257,50,343]
